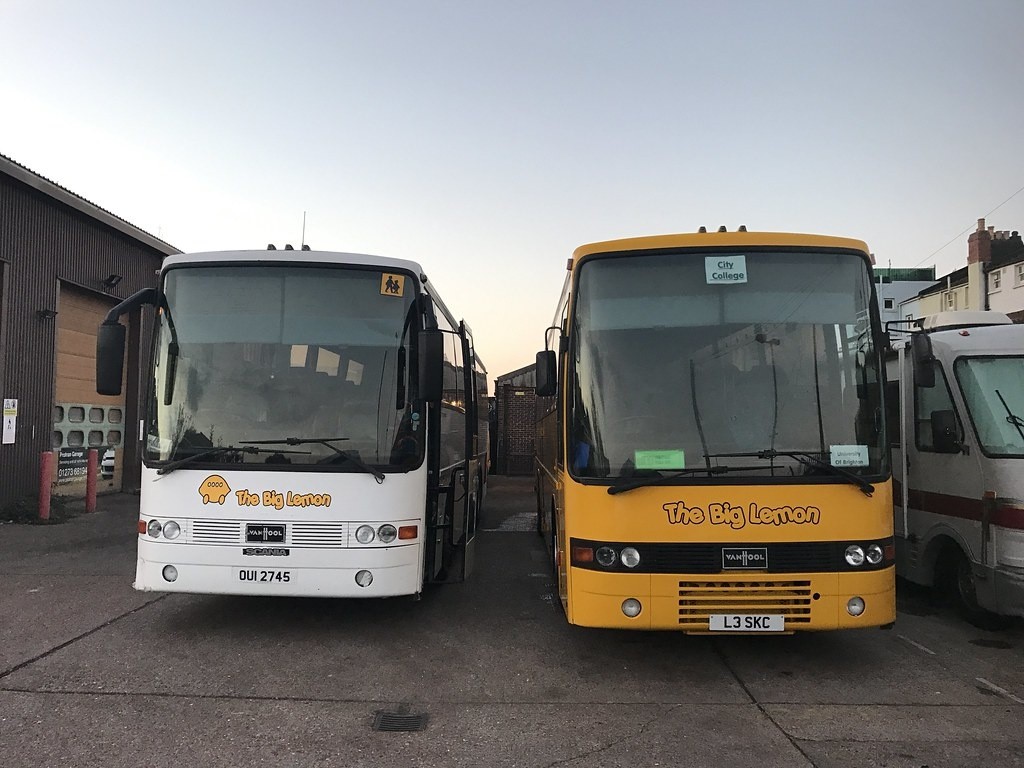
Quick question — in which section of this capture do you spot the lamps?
[102,275,122,286]
[37,309,58,320]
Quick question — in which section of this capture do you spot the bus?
[533,224,903,637]
[95,242,490,603]
[835,310,1024,634]
